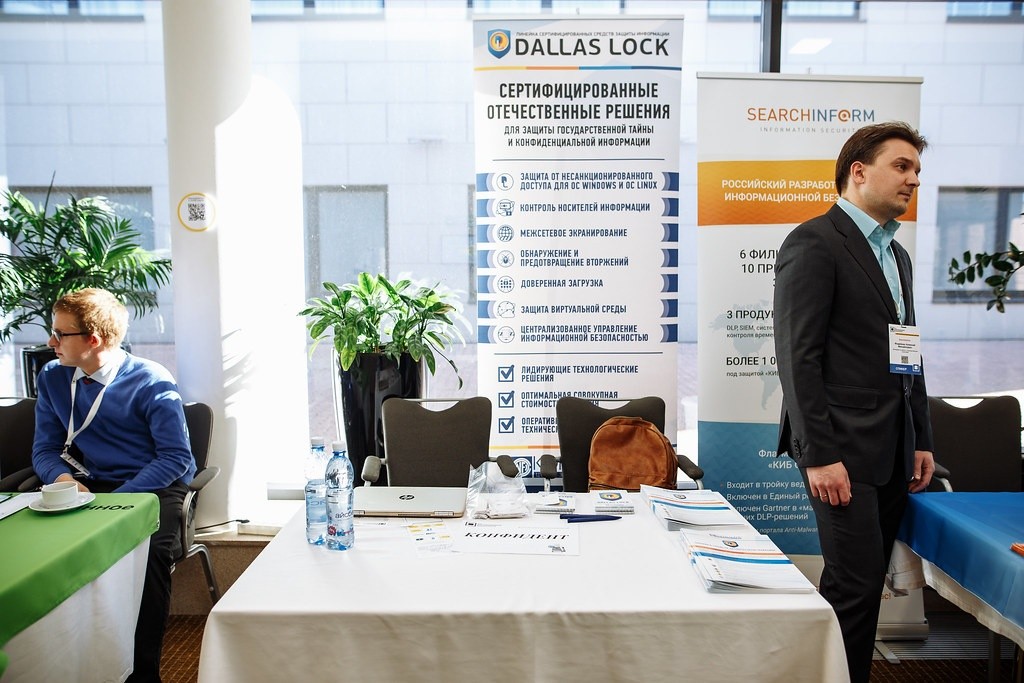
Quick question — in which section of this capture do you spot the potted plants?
[0,169,172,400]
[297,273,465,486]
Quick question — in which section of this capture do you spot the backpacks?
[589,415,677,492]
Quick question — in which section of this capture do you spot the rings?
[819,494,828,498]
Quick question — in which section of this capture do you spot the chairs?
[536,395,705,491]
[360,396,519,487]
[0,397,222,605]
[923,394,1024,493]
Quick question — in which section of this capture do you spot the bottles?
[325,441,354,550]
[305,437,328,545]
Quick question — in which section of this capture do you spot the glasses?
[51,328,86,342]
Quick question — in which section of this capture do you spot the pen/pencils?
[560,513,622,523]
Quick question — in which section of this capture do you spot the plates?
[29,491,96,511]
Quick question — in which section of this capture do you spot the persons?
[773,121,937,683]
[31,287,198,683]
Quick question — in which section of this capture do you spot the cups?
[41,481,79,508]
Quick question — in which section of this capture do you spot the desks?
[895,492,1024,683]
[196,493,854,683]
[0,491,160,683]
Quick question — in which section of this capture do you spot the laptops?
[352,486,468,517]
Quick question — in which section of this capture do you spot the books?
[639,483,818,595]
[590,489,635,515]
[533,490,577,515]
